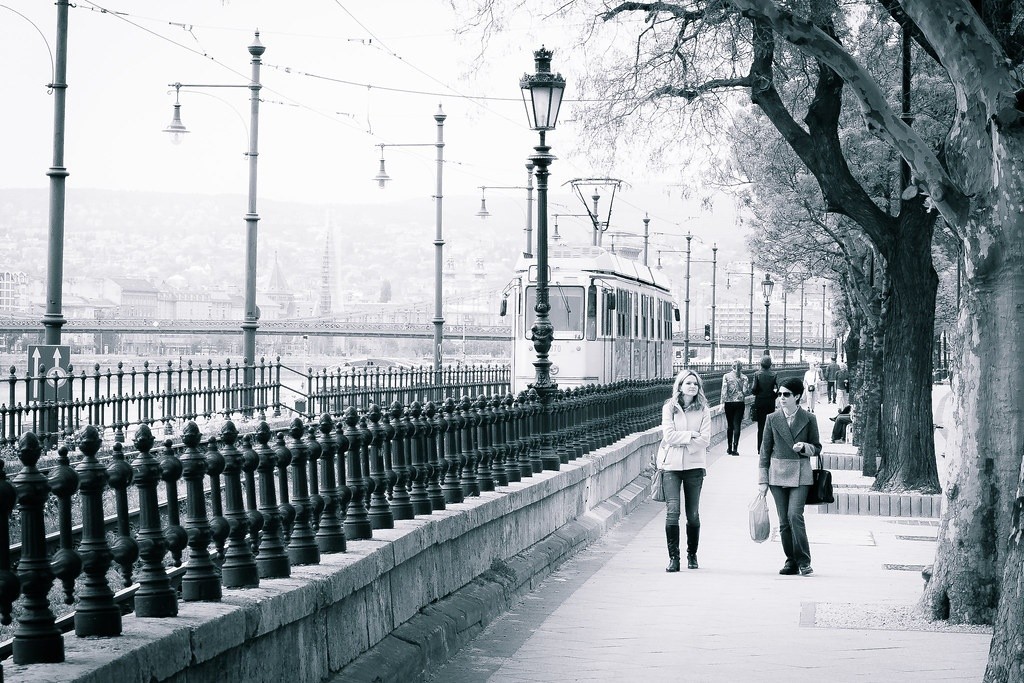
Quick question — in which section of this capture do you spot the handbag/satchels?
[808,385,815,391]
[748,493,770,544]
[805,444,834,505]
[750,404,758,421]
[651,470,666,502]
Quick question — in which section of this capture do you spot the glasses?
[777,392,797,397]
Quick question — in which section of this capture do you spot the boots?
[686,524,700,569]
[666,525,680,572]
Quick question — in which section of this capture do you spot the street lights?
[656,229,694,369]
[517,42,567,398]
[608,211,651,266]
[372,101,448,408]
[160,27,266,424]
[724,257,756,369]
[781,273,827,363]
[550,187,601,247]
[760,269,775,356]
[474,153,535,255]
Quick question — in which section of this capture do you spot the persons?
[758,377,821,574]
[716,361,749,456]
[657,370,711,571]
[824,406,851,443]
[803,361,823,412]
[824,357,848,410]
[750,356,779,454]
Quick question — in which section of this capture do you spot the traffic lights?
[705,324,711,341]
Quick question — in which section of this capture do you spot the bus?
[499,265,681,407]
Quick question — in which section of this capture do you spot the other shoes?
[727,449,732,454]
[779,560,799,574]
[829,418,836,421]
[800,565,814,575]
[732,451,739,455]
